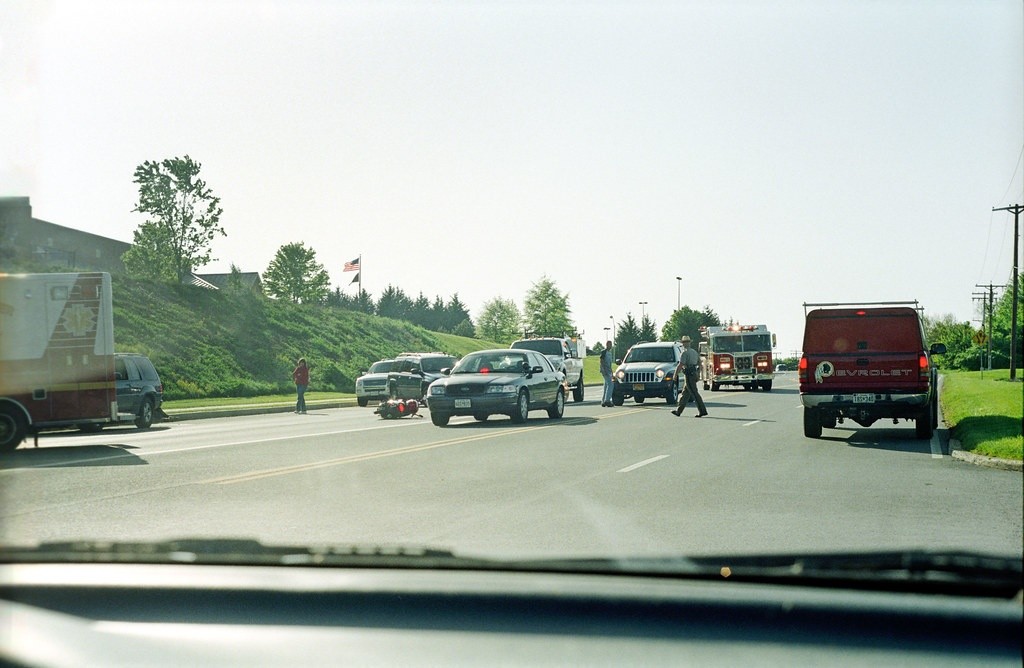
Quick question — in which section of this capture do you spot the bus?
[775,363,789,371]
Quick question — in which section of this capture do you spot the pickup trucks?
[499,333,585,403]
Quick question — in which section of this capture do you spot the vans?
[798,299,948,440]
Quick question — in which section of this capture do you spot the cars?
[427,347,569,426]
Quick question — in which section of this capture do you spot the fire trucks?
[697,323,777,392]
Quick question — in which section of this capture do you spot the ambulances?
[0,271,121,453]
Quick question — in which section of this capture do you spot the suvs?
[612,340,695,406]
[385,351,465,409]
[356,357,395,407]
[73,352,164,432]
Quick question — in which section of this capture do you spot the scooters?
[373,394,424,420]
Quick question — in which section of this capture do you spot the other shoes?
[671,410,680,417]
[695,412,708,417]
[298,411,307,415]
[294,410,298,415]
[601,401,615,408]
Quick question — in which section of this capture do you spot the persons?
[671,335,708,418]
[599,340,614,407]
[291,358,309,414]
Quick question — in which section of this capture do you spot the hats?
[678,336,694,343]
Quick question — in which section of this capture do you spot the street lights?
[603,327,611,341]
[610,316,615,363]
[639,301,648,330]
[676,276,682,312]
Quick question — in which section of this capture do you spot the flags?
[343,258,359,272]
[348,273,359,286]
